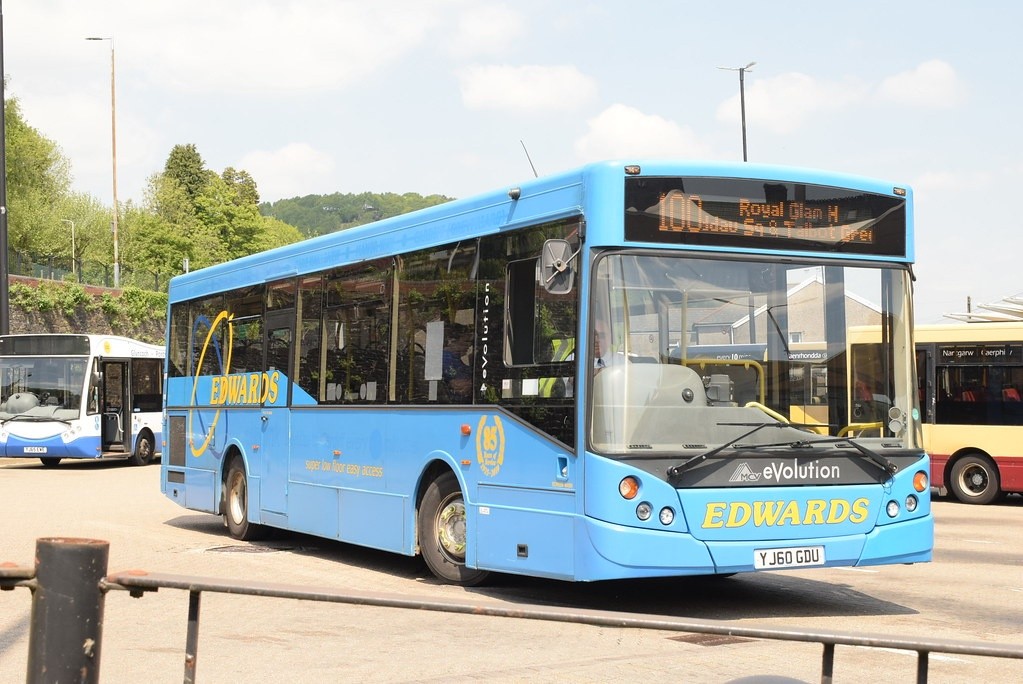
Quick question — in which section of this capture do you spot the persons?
[432,320,477,397]
[558,314,629,396]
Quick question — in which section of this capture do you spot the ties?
[598,359,606,368]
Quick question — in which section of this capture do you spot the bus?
[0,334,167,468]
[846,321,1023,504]
[159,157,933,587]
[668,342,830,436]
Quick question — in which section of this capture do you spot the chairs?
[812,396,828,404]
[538,337,575,397]
[857,381,1023,401]
[179,338,454,404]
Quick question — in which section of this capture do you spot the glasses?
[595,330,609,341]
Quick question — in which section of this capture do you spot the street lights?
[61,219,75,274]
[86,36,118,287]
[712,298,818,356]
